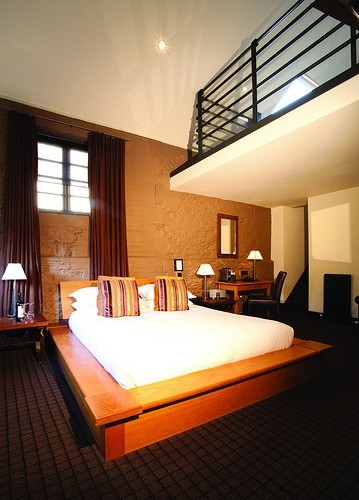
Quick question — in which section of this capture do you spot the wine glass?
[19,303,29,324]
[26,303,35,324]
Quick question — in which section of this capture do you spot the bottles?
[16,293,24,322]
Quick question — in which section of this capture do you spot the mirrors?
[217,213,239,258]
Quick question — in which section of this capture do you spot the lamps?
[1,263,27,318]
[247,250,264,282]
[196,264,215,299]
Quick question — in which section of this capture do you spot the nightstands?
[189,298,233,312]
[0,314,49,364]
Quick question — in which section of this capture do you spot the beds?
[44,278,334,463]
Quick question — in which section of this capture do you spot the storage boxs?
[209,289,226,298]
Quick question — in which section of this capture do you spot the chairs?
[247,271,287,321]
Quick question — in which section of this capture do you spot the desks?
[215,280,274,314]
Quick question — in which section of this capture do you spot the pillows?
[68,275,197,318]
